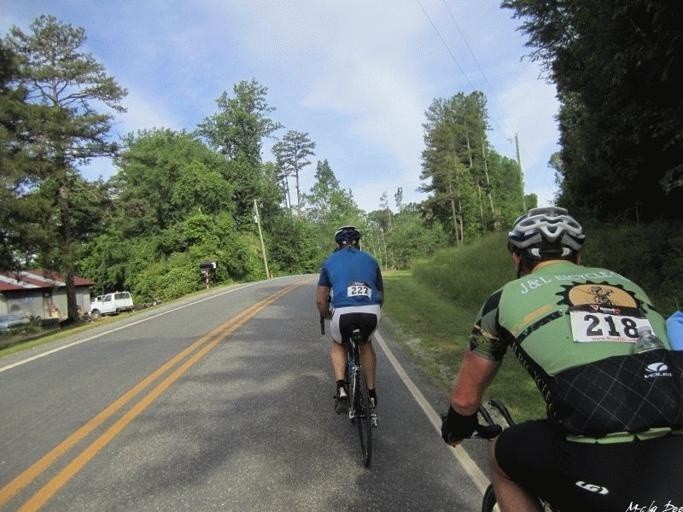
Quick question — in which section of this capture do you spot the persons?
[441,204,683,511]
[317,225,389,426]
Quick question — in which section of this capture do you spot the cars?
[0,314,30,335]
[88,291,134,319]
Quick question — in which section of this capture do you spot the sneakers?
[336,379,349,401]
[368,395,378,409]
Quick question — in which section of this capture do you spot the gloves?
[440,405,479,446]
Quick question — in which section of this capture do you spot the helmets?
[334,225,361,244]
[506,206,586,259]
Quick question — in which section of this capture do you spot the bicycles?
[320,302,379,468]
